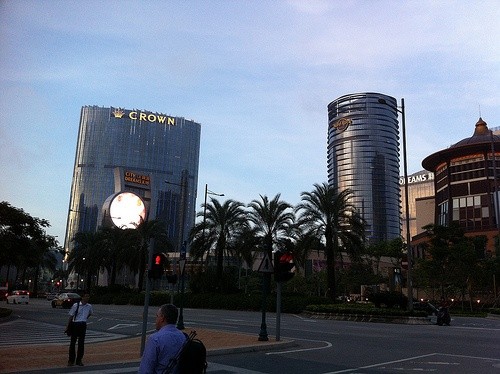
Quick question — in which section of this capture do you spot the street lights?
[164,179,186,248]
[200,192,224,272]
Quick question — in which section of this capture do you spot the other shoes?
[68,362,73,366]
[76,362,83,366]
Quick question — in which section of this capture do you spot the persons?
[137,304,187,374]
[64,292,93,366]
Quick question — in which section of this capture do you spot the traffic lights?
[275,250,296,283]
[57,282,60,285]
[152,254,164,279]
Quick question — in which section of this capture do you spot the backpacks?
[161,333,207,374]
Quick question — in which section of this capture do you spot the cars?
[51,292,83,308]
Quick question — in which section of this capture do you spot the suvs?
[7,290,30,304]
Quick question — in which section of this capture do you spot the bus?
[0,281,8,300]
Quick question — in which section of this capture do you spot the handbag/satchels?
[67,321,79,336]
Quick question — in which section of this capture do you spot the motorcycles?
[432,306,450,326]
